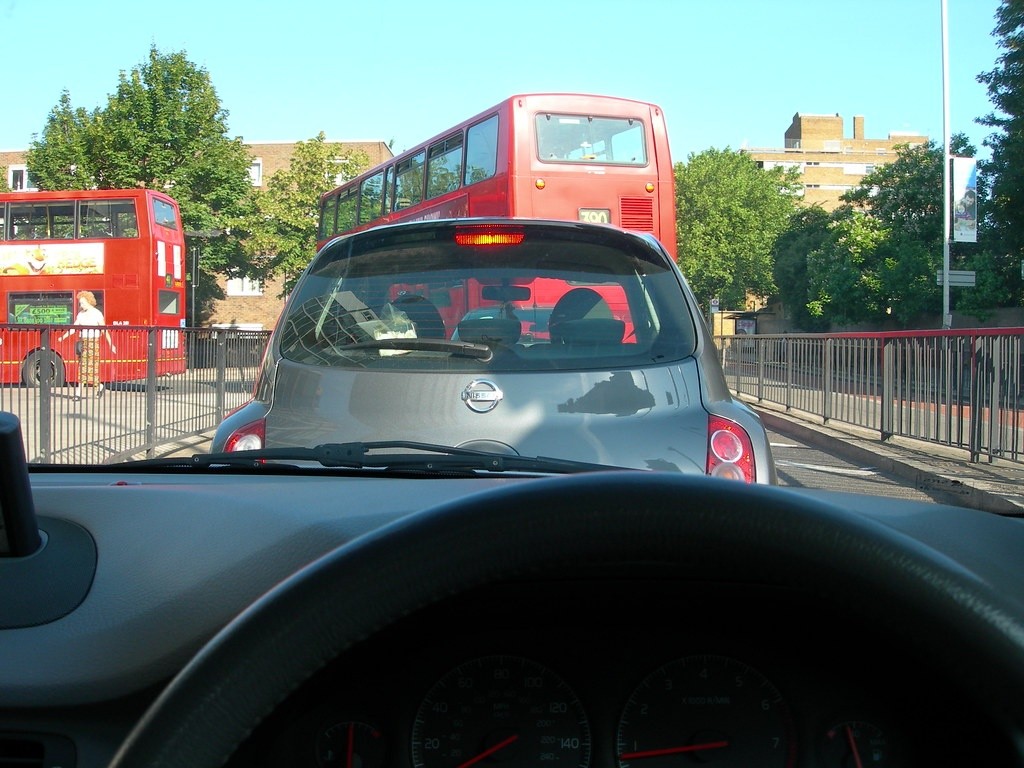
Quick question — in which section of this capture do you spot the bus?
[1,184,188,391]
[306,87,677,357]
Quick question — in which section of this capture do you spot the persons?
[57,290,117,402]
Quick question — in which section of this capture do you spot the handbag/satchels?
[76,341,84,355]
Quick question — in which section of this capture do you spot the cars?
[211,208,780,499]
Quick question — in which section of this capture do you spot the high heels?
[97,384,105,399]
[72,391,82,401]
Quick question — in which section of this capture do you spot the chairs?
[7,311,72,325]
[355,287,626,354]
